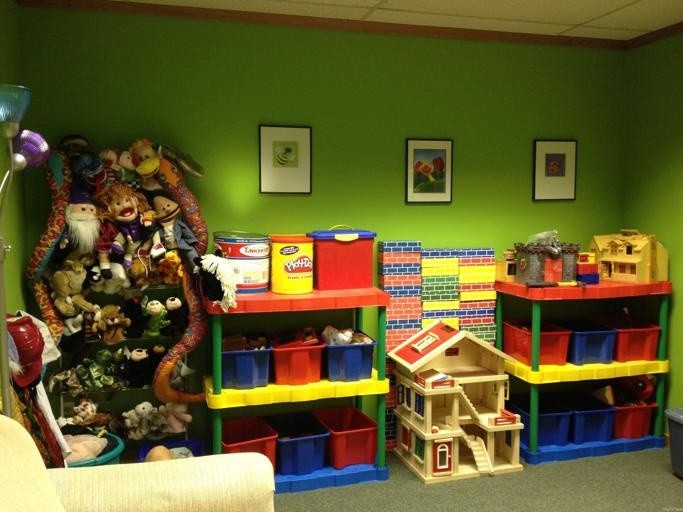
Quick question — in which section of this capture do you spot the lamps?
[0,82,51,418]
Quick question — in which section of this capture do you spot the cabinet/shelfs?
[59,284,188,442]
[197,279,390,495]
[494,278,672,463]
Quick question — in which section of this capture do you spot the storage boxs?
[503,319,574,366]
[597,317,660,362]
[550,319,616,365]
[222,326,376,389]
[306,224,377,291]
[505,401,659,450]
[310,406,377,470]
[209,415,279,474]
[262,412,332,476]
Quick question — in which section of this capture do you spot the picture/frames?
[258,124,312,194]
[406,138,452,203]
[533,139,577,200]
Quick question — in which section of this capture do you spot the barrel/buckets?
[67,429,127,467]
[271,233,314,295]
[210,229,270,294]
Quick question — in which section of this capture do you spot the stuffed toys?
[222,325,373,352]
[25,134,209,441]
[24,134,209,440]
[592,375,657,408]
